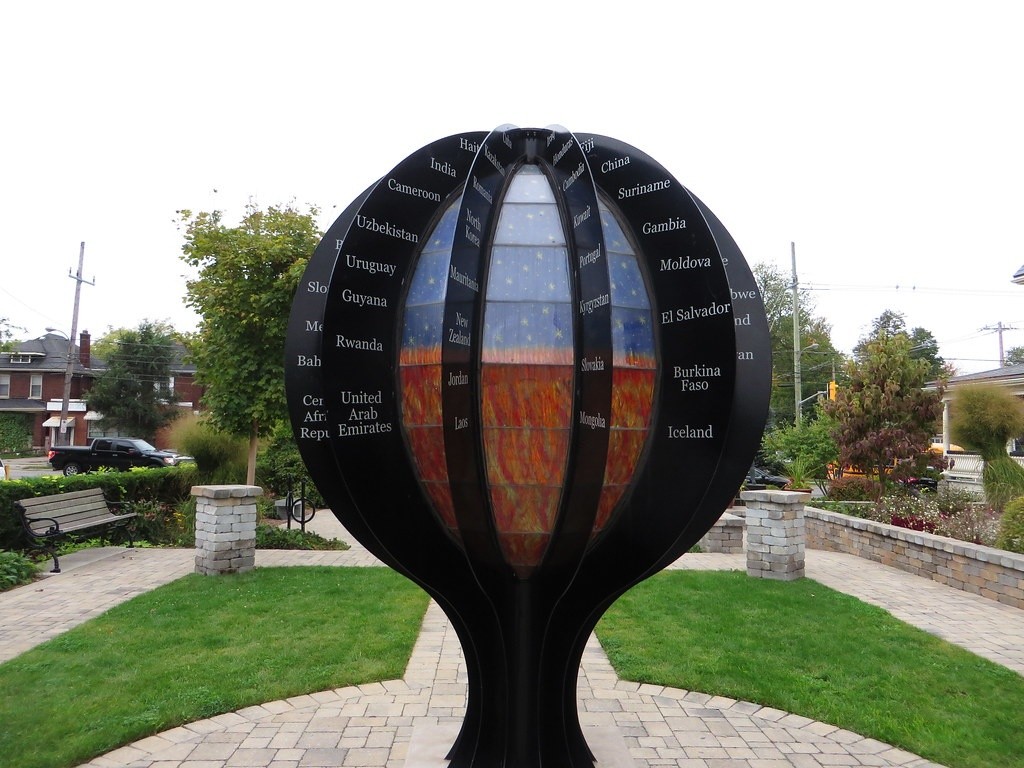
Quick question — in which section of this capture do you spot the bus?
[826,434,965,485]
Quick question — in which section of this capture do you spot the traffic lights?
[829,380,840,402]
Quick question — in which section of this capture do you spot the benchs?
[2,487,138,573]
[275,497,307,520]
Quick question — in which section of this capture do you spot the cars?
[743,466,791,491]
[888,458,938,495]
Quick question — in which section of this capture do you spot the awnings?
[84,411,109,421]
[0,398,46,412]
[42,415,76,428]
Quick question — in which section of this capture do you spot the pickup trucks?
[47,436,195,479]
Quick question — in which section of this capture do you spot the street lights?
[44,327,77,446]
[794,344,820,425]
